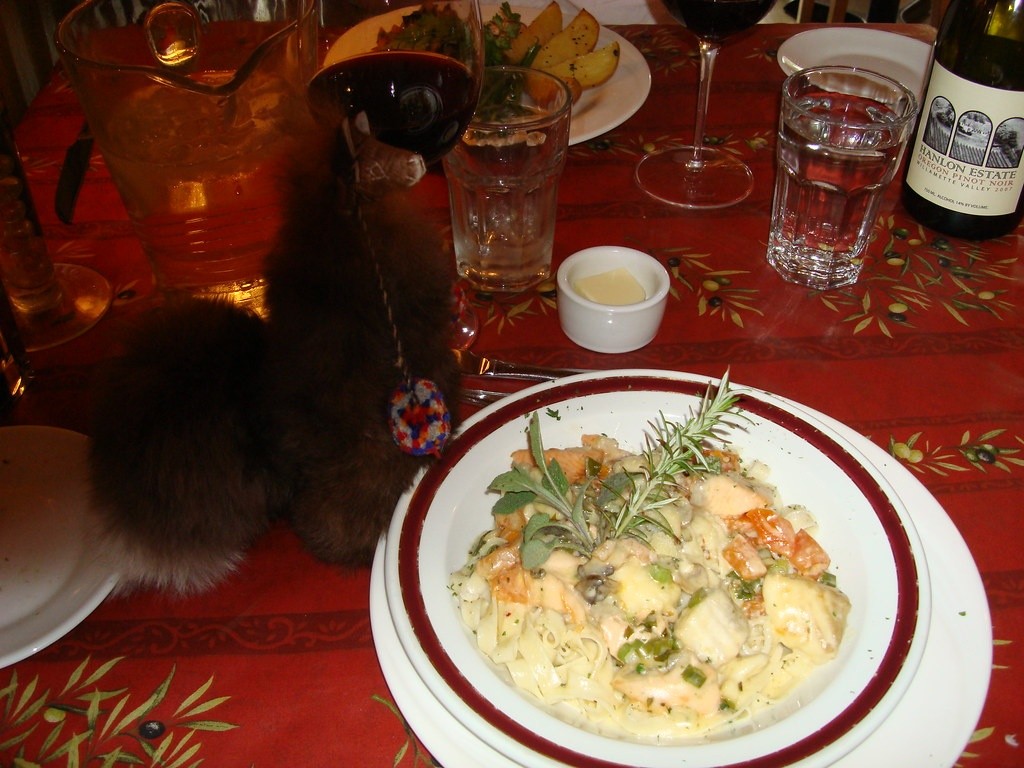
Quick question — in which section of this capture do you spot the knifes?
[53,120,94,225]
[451,349,587,381]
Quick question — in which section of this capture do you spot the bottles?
[902,0,1024,241]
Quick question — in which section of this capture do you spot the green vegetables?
[483,365,756,570]
[399,0,543,117]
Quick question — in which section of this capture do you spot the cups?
[0,95,113,353]
[765,66,919,291]
[555,245,671,355]
[0,277,35,418]
[444,64,572,293]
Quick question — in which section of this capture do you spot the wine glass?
[308,0,485,350]
[635,0,776,209]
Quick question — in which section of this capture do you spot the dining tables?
[1,16,1023,766]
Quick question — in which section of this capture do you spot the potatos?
[504,2,619,101]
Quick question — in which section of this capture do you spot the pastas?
[451,568,619,729]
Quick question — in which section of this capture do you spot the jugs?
[55,0,317,319]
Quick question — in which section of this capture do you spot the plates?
[0,426,128,669]
[323,0,652,147]
[776,27,932,112]
[369,368,994,768]
